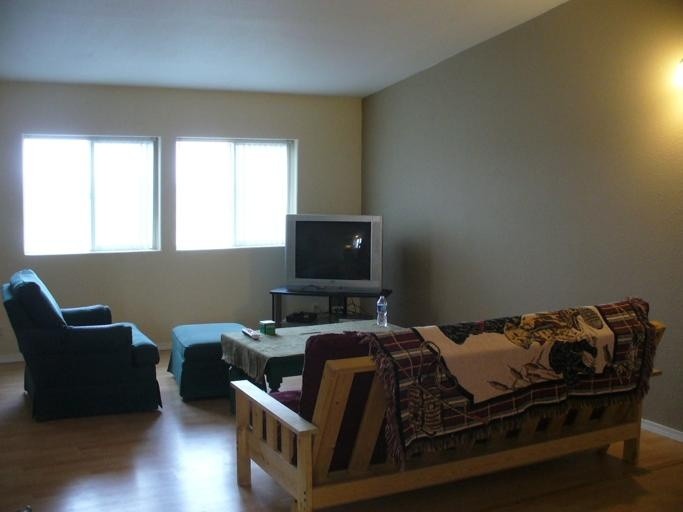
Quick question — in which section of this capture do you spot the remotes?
[242,328,259,339]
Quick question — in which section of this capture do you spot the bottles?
[376,295,388,328]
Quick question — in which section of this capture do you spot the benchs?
[230,319,667,512]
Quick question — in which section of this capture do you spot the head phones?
[287,311,317,323]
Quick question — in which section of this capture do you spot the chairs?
[2,283,163,421]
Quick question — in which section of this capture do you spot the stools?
[167,323,247,402]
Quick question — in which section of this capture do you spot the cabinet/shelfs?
[270,286,392,328]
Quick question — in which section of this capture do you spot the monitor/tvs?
[286,214,383,294]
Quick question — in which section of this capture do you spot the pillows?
[10,268,68,330]
